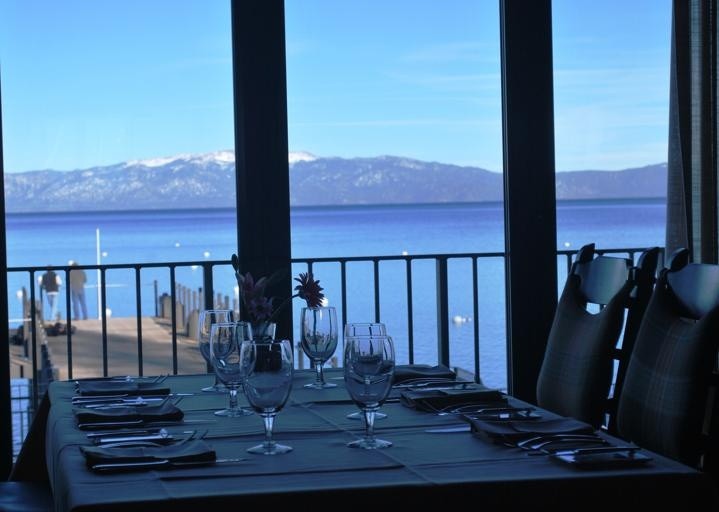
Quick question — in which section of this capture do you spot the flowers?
[231,252,326,336]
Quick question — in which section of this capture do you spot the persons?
[68,260,89,321]
[38,263,63,322]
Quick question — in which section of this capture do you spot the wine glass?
[342,335,396,451]
[239,337,294,454]
[210,321,258,418]
[300,306,339,389]
[197,309,236,393]
[342,322,389,420]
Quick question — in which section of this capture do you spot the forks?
[391,374,653,467]
[68,368,249,473]
